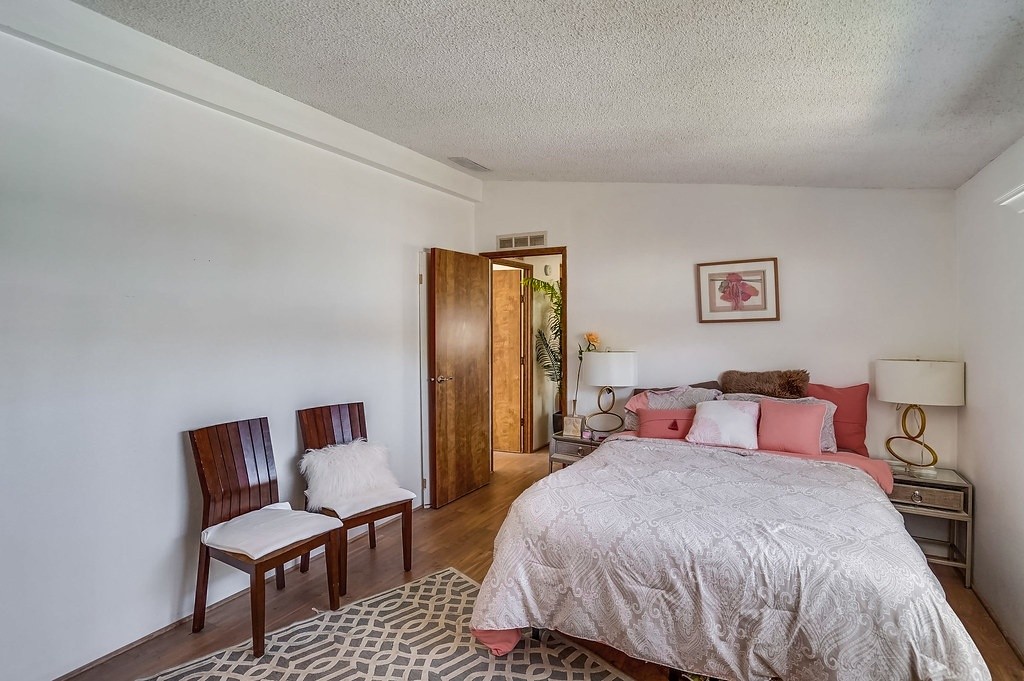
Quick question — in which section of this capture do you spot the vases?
[563,400,585,438]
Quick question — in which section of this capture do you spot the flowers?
[574,327,602,402]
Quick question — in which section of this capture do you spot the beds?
[468,380,996,681]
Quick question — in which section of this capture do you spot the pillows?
[720,369,811,399]
[298,438,399,512]
[758,399,827,456]
[685,401,760,451]
[623,388,722,432]
[636,407,696,439]
[807,383,870,458]
[716,393,838,453]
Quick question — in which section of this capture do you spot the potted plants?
[522,275,563,434]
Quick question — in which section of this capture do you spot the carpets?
[132,567,637,681]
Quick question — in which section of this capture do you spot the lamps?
[581,351,638,433]
[873,358,965,481]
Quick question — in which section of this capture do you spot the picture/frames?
[695,257,781,324]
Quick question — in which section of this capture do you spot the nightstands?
[548,431,602,475]
[882,465,974,590]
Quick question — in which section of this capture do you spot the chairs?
[189,417,341,658]
[296,402,416,598]
